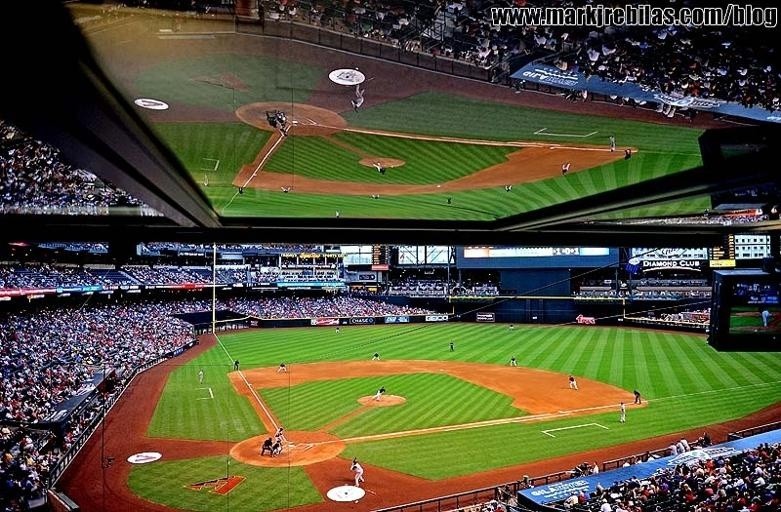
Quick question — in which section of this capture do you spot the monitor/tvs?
[699,124,780,209]
[707,270,781,353]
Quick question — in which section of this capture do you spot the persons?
[1,0,780,242]
[0,242,781,512]
